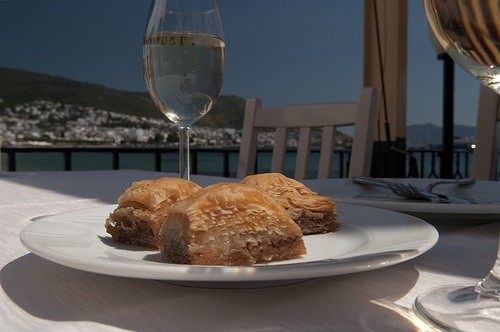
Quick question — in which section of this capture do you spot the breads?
[105,178,203,250]
[158,182,308,266]
[240,172,341,233]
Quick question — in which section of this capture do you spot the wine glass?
[141,0,224,180]
[416,0,500,331]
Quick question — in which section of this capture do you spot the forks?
[389,178,475,199]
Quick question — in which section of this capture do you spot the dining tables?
[0,160,500,332]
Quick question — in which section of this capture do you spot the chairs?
[235,87,381,179]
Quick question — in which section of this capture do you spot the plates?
[20,202,439,289]
[300,178,500,223]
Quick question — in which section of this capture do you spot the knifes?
[352,175,477,204]
